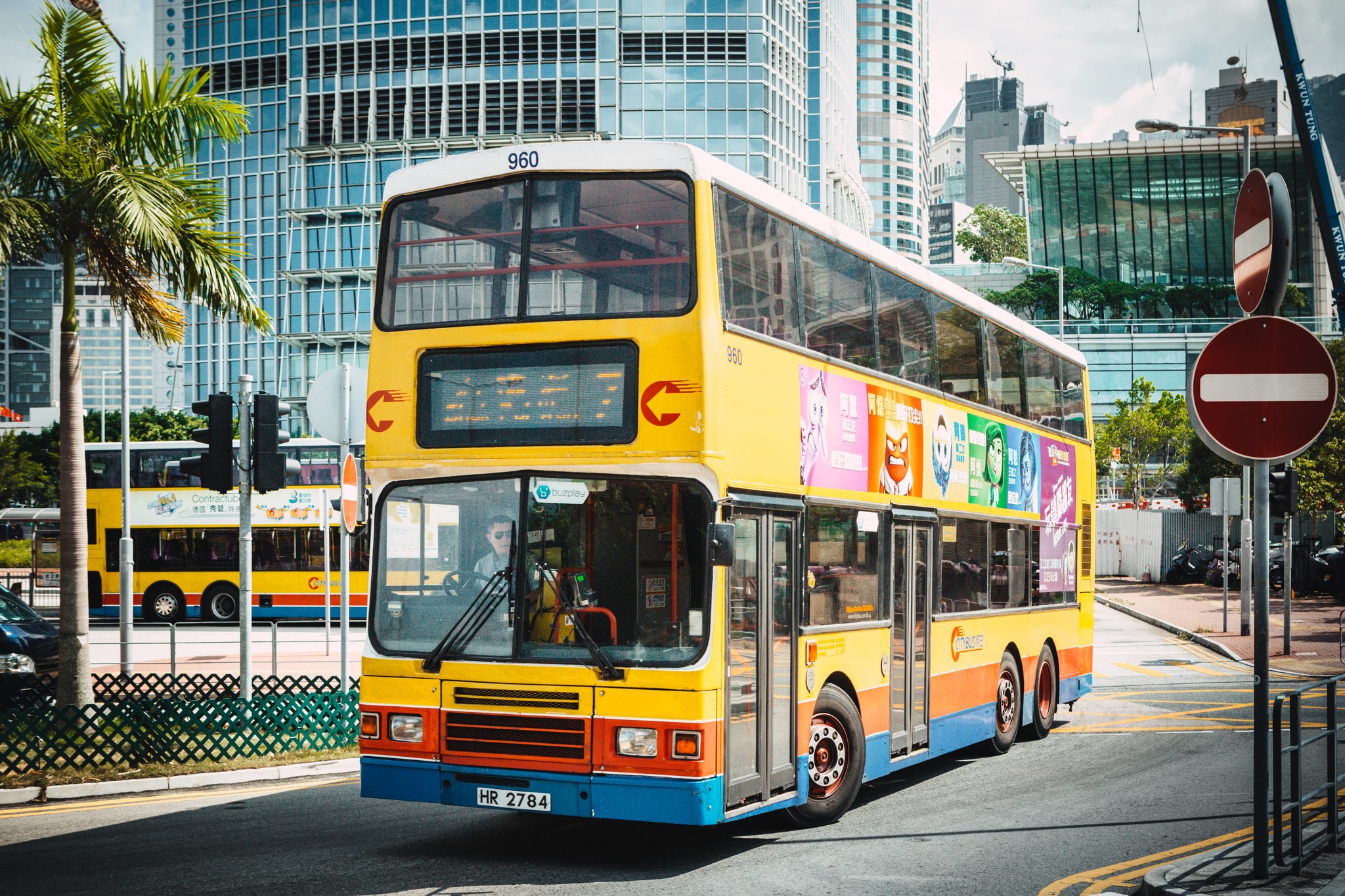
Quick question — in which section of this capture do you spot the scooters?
[1206,534,1345,605]
[1166,538,1213,585]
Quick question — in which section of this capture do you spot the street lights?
[1132,116,1252,172]
[1001,256,1064,342]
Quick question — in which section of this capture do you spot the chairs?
[895,559,1026,612]
[108,469,173,488]
[727,316,1085,436]
[288,469,334,484]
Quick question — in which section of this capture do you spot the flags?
[0,405,22,422]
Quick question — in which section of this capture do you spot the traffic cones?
[1142,565,1154,584]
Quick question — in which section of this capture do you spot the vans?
[0,584,58,709]
[0,524,25,543]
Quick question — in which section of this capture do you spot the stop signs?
[1233,168,1273,314]
[1190,316,1338,461]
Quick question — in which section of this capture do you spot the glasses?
[490,530,512,539]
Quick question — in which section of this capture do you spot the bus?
[84,441,460,626]
[358,138,1096,828]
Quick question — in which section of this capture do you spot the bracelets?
[524,598,534,607]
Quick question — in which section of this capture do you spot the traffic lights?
[253,389,301,495]
[1269,469,1299,517]
[180,391,234,495]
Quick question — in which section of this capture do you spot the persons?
[1181,307,1191,322]
[1152,308,1160,317]
[1127,312,1138,334]
[1159,321,1174,333]
[1057,316,1075,334]
[473,515,539,621]
[1226,313,1236,326]
